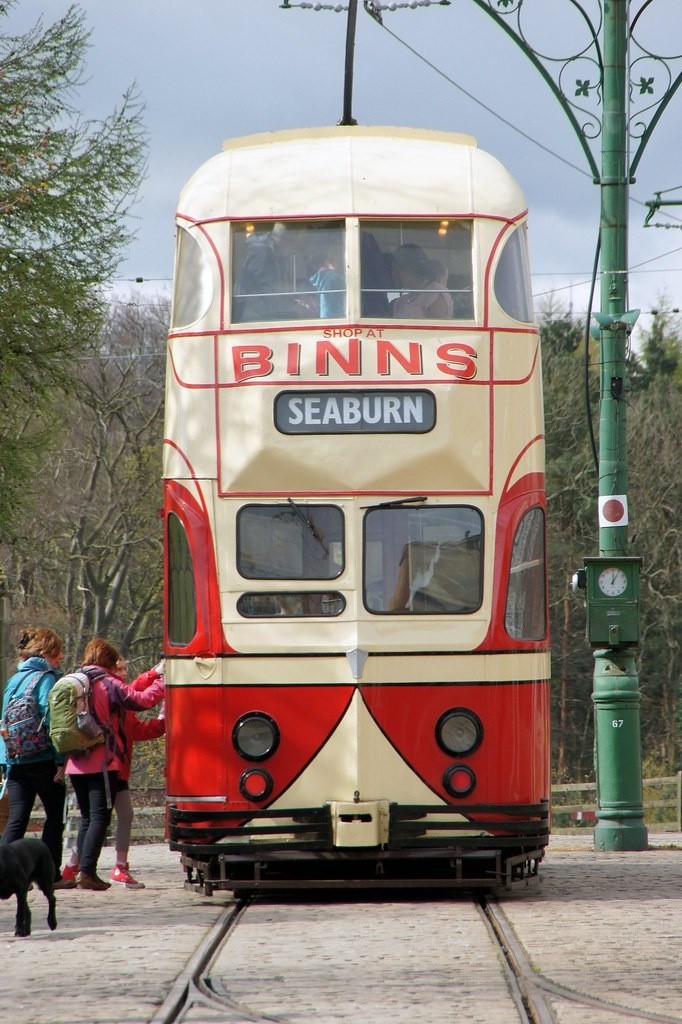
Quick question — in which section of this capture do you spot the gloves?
[157,701,165,721]
[155,658,165,675]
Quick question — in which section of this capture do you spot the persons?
[299,222,391,320]
[0,627,78,889]
[233,218,320,321]
[384,541,410,613]
[387,242,474,320]
[62,653,166,889]
[65,639,164,891]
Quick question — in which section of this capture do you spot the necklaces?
[407,279,429,303]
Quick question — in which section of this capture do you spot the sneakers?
[110,862,145,888]
[76,869,112,890]
[52,879,76,889]
[27,883,33,891]
[61,863,80,881]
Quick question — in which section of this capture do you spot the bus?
[157,125,553,902]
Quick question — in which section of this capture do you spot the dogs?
[0,838,57,938]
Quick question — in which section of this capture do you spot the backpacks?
[1,670,57,761]
[48,664,116,755]
